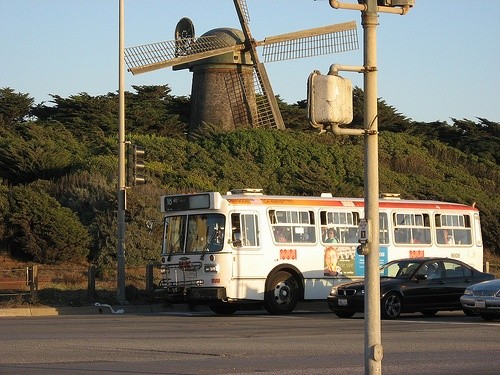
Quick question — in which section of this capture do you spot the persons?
[325,246,343,276]
[441,228,454,245]
[424,265,437,279]
[326,229,337,244]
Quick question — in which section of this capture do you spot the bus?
[159,188,482,314]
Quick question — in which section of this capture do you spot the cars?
[460,278,500,322]
[327,256,497,320]
